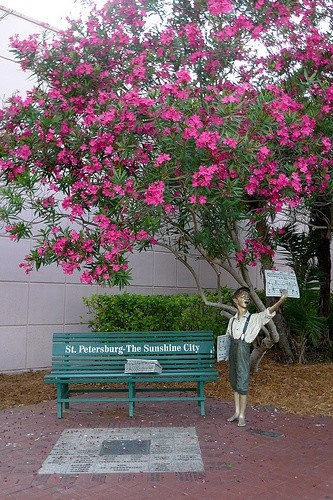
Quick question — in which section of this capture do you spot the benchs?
[45,328,220,421]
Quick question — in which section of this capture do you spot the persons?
[222,285,289,428]
